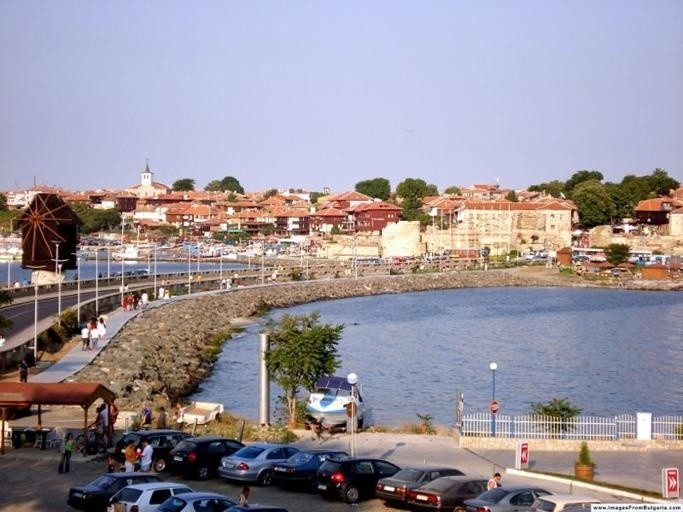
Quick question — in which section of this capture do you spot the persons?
[222,269,240,291]
[72,272,78,284]
[81,312,108,352]
[18,359,29,383]
[13,278,32,289]
[57,433,77,474]
[123,286,170,312]
[237,487,250,505]
[88,403,184,474]
[487,473,501,491]
[95,271,146,282]
[303,414,327,446]
[111,497,127,512]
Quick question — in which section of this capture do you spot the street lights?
[25,264,47,366]
[51,239,64,283]
[489,362,498,437]
[51,257,70,330]
[346,372,359,460]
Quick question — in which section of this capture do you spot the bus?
[622,251,653,264]
[572,248,613,263]
[655,254,683,270]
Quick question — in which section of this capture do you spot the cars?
[376,465,466,506]
[218,443,302,487]
[524,251,534,260]
[224,505,290,512]
[570,256,590,265]
[275,449,350,494]
[527,494,602,512]
[408,474,503,511]
[153,492,241,512]
[463,486,553,512]
[67,471,164,512]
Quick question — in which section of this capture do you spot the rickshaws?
[172,400,224,427]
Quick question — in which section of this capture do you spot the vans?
[314,457,402,504]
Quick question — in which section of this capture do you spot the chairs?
[46,426,64,448]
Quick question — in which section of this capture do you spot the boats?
[305,376,365,431]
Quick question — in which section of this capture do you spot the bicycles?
[74,423,94,449]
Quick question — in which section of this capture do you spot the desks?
[12,427,55,450]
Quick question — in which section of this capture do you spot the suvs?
[115,429,192,472]
[107,482,195,511]
[169,436,246,481]
